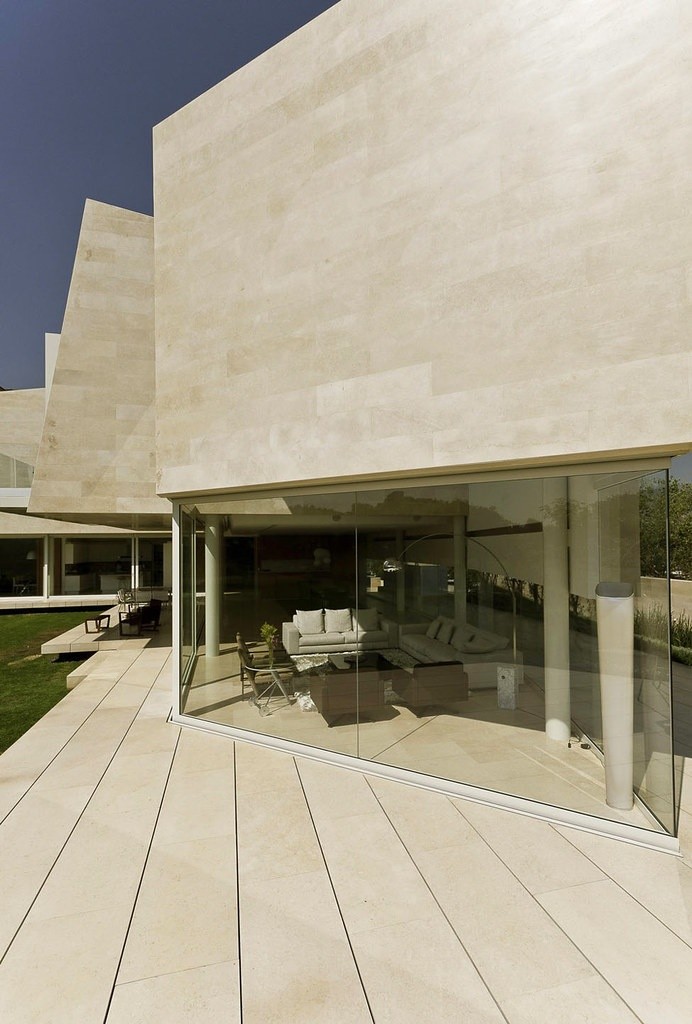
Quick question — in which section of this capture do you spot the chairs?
[12,575,36,595]
[117,588,134,612]
[235,632,294,697]
[392,661,469,719]
[309,662,384,726]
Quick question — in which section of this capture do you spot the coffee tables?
[329,652,396,681]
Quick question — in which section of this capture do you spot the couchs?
[282,615,398,655]
[398,616,524,690]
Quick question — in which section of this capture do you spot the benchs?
[86,614,111,633]
[134,585,171,609]
[135,599,163,632]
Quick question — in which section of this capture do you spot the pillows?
[324,607,353,633]
[494,633,512,650]
[463,638,495,654]
[436,623,454,644]
[351,607,379,632]
[451,625,474,649]
[426,619,441,639]
[296,609,325,636]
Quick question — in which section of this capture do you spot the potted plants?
[260,622,278,669]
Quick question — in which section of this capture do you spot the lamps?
[27,551,37,560]
[382,532,517,662]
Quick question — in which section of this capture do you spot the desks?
[119,613,143,636]
[245,657,299,717]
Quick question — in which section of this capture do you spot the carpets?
[287,649,421,711]
